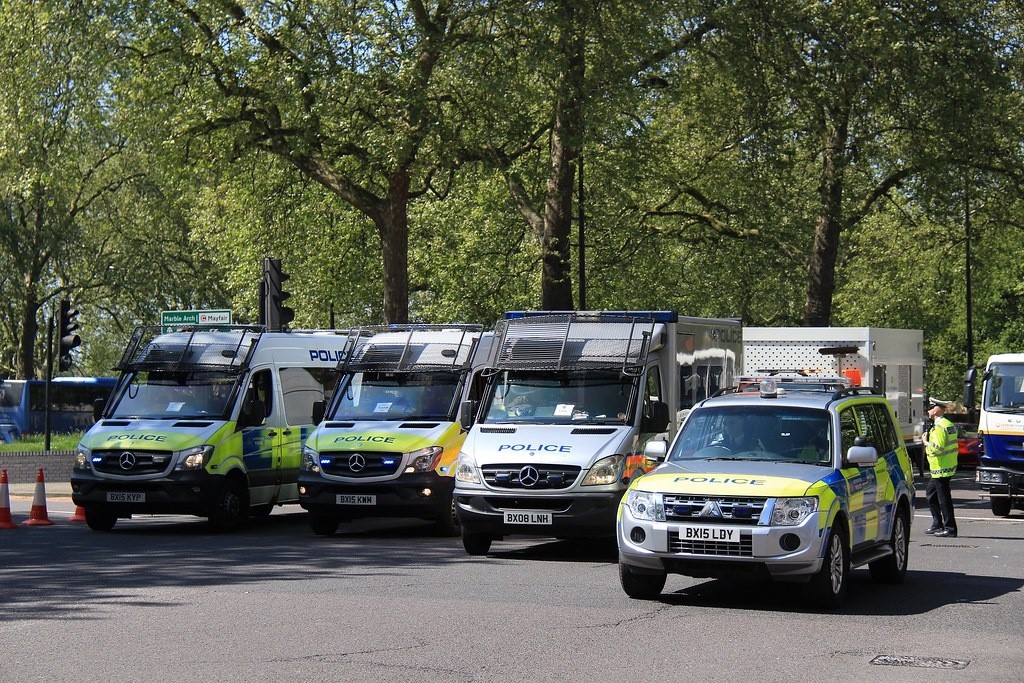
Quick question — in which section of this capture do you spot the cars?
[954,422,980,470]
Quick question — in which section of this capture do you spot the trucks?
[963,353,1023,516]
[452,313,745,555]
[298,322,498,537]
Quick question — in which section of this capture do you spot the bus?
[0,376,138,443]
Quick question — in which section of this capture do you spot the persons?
[922,396,958,537]
[711,412,765,453]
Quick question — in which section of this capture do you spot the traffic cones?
[21,467,55,525]
[0,468,20,529]
[70,505,87,522]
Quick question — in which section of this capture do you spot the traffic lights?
[59,298,81,372]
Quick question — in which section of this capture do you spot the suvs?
[616,366,918,609]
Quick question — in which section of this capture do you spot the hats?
[926,396,951,411]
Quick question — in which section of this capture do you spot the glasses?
[723,421,738,426]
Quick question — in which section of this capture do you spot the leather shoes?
[925,527,938,534]
[934,528,958,537]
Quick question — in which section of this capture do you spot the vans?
[70,322,373,534]
